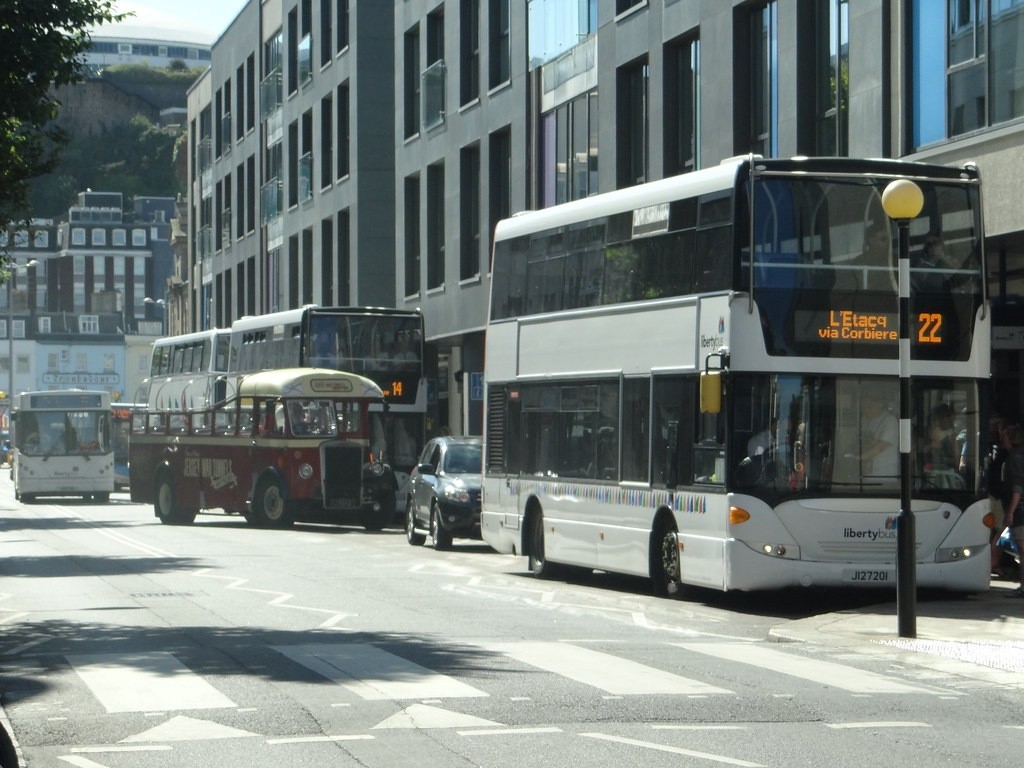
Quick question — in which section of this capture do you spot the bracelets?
[943,254,951,261]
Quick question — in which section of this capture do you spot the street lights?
[880,179,924,640]
[6,259,43,445]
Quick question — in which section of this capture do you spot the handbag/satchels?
[996,525,1020,557]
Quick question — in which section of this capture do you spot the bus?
[10,389,116,502]
[479,151,992,599]
[126,365,403,529]
[224,304,435,518]
[148,326,230,428]
[109,402,152,492]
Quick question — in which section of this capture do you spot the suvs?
[404,433,489,552]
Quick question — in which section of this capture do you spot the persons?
[744,390,1024,599]
[26,423,50,445]
[909,230,969,294]
[308,332,419,371]
[852,224,900,294]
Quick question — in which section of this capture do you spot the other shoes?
[1004,587,1024,598]
